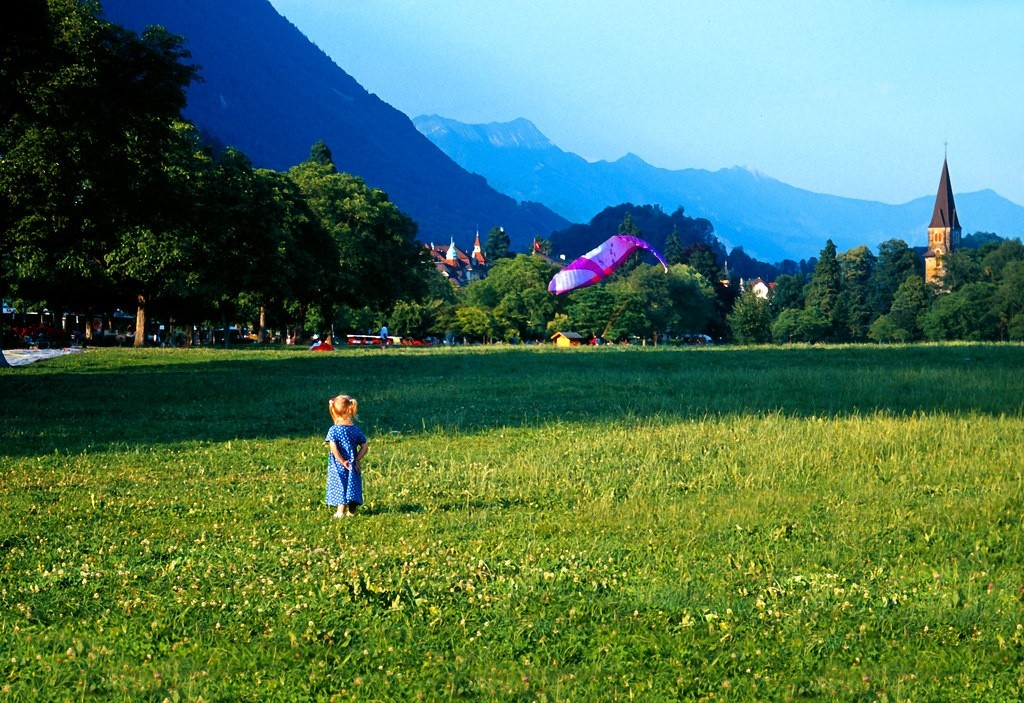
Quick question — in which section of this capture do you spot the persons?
[325,395,368,520]
[380,320,389,350]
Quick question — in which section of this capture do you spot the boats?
[309,338,334,351]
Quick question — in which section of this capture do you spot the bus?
[212,327,254,344]
[347,335,403,346]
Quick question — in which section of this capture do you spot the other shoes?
[346,513,354,517]
[334,513,344,518]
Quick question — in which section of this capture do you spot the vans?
[680,334,714,347]
